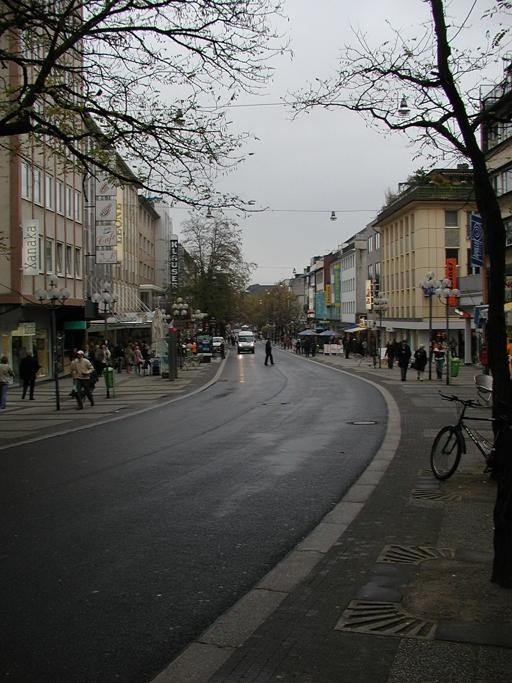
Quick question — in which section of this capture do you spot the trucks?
[237,331,255,353]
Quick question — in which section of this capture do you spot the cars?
[230,328,257,343]
[212,336,226,352]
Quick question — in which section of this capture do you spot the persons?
[264,336,275,366]
[413,343,427,381]
[302,336,368,359]
[18,349,41,400]
[507,338,512,379]
[70,349,94,410]
[432,343,446,379]
[383,338,403,369]
[396,337,411,381]
[294,339,301,355]
[82,340,152,375]
[1,354,15,409]
[181,337,198,358]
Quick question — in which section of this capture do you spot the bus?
[241,325,249,330]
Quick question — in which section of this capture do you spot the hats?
[77,350,85,355]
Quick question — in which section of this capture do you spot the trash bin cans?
[451,358,460,376]
[103,367,113,387]
[316,345,320,352]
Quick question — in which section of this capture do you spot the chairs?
[474,374,493,406]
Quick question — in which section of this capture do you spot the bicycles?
[429,388,496,486]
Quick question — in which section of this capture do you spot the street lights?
[36,270,70,410]
[171,296,189,370]
[373,291,389,368]
[416,271,442,381]
[192,308,209,355]
[436,277,460,385]
[90,280,118,398]
